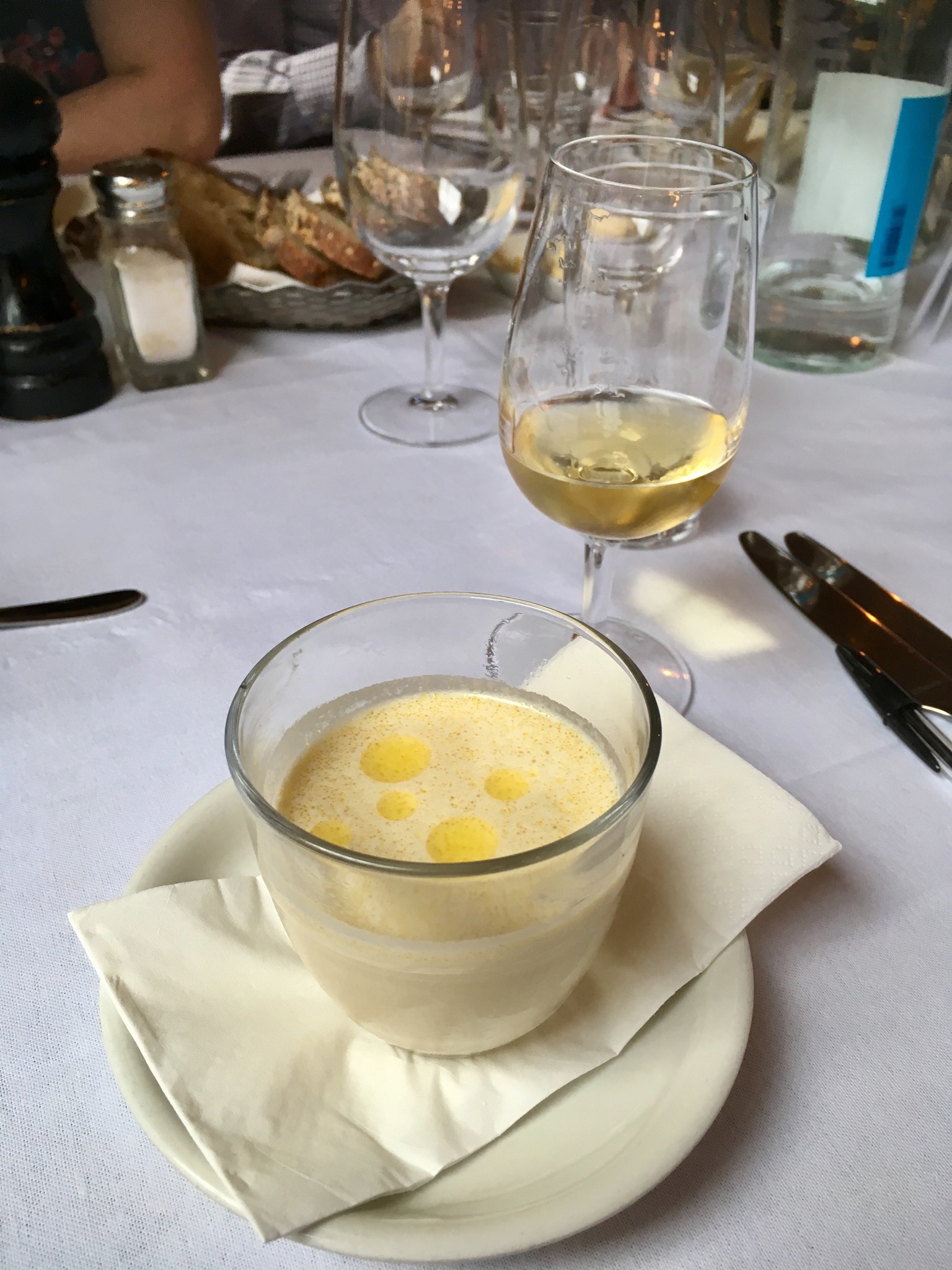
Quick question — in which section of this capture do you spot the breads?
[138,148,488,286]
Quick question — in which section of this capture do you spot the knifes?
[0,588,145,628]
[738,530,951,718]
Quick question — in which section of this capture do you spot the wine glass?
[331,0,772,450]
[483,131,758,718]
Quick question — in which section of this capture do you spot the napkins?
[45,626,855,1258]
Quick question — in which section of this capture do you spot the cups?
[222,591,663,1056]
[563,163,774,549]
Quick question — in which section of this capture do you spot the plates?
[486,259,522,302]
[94,782,756,1264]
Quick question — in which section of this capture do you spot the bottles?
[731,0,951,377]
[88,155,213,394]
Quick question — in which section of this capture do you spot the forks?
[144,146,311,200]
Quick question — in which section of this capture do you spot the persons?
[0,0,223,170]
[217,0,489,150]
